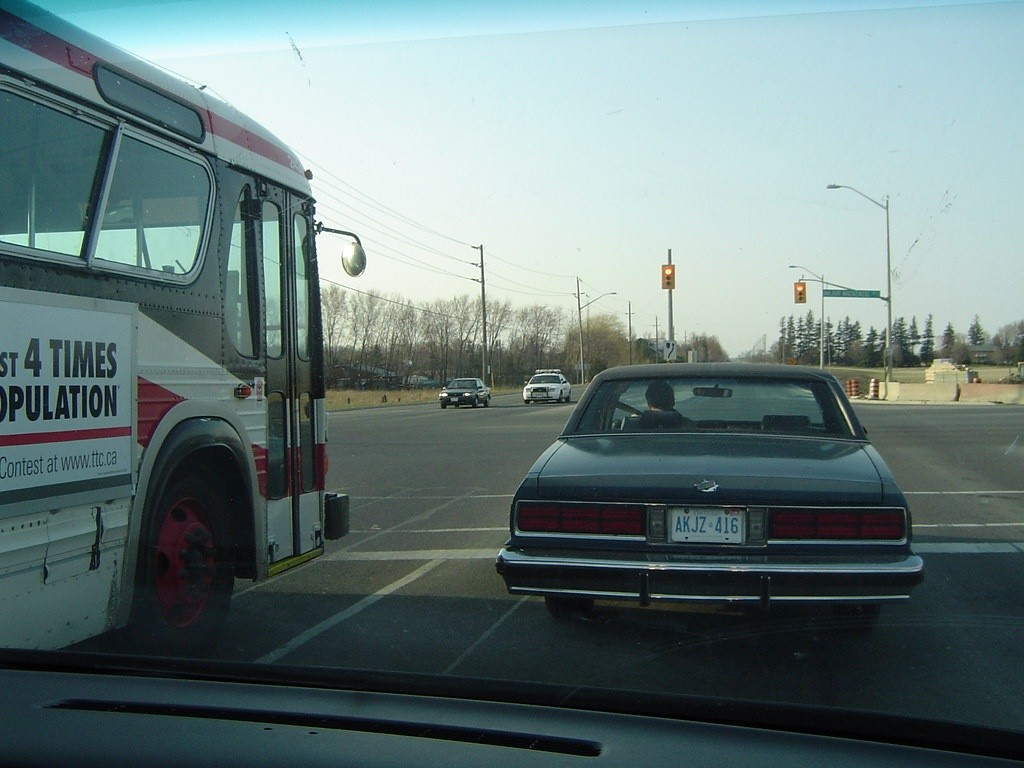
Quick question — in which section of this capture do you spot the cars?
[490,361,926,627]
[438,378,492,413]
[520,369,571,404]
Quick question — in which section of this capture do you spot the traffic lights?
[794,281,809,304]
[662,264,676,290]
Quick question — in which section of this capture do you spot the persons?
[630,381,678,417]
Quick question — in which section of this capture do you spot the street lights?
[577,291,619,385]
[790,265,825,370]
[826,184,892,383]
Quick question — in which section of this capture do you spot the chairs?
[760,415,810,432]
[621,409,699,430]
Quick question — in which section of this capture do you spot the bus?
[1,2,367,664]
